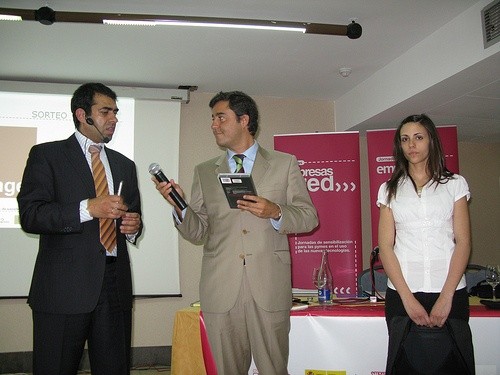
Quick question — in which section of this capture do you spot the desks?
[170,300,500,375]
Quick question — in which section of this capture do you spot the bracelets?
[272,207,282,220]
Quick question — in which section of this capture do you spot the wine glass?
[485,264,500,300]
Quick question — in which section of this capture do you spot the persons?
[16,83,144,375]
[377,115,476,375]
[151,91,320,375]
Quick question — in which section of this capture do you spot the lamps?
[0,6,362,40]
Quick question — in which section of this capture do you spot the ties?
[232,155,245,173]
[88,145,118,253]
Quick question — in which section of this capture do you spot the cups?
[312,265,326,289]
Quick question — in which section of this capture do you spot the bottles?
[318,250,333,303]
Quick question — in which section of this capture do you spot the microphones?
[148,163,187,210]
[85,118,109,144]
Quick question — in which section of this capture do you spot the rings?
[111,209,114,214]
